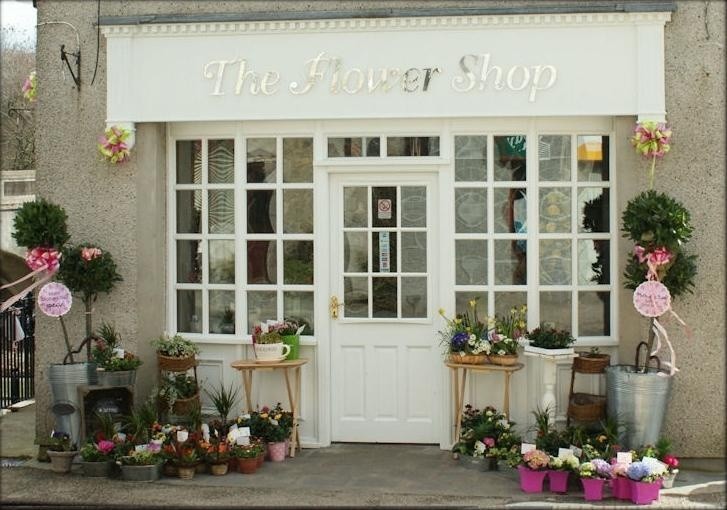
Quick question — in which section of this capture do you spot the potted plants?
[9,195,144,447]
[602,189,699,449]
[151,333,198,368]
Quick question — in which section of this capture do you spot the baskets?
[164,390,201,416]
[449,348,490,365]
[574,352,612,375]
[157,351,197,373]
[488,352,521,367]
[570,393,609,423]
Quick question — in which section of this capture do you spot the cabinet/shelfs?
[569,352,608,438]
[156,361,201,422]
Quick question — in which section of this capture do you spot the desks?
[446,360,518,455]
[232,358,307,459]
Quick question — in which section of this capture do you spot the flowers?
[39,406,291,479]
[450,399,680,507]
[435,299,534,371]
